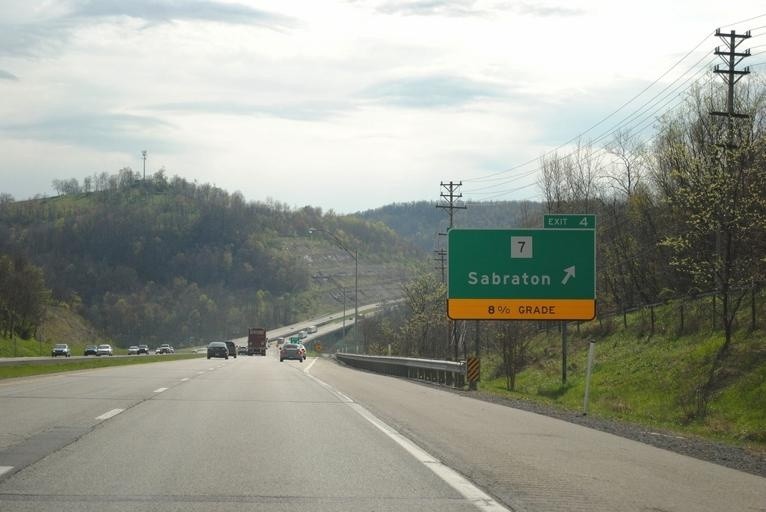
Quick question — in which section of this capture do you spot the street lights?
[308,228,358,336]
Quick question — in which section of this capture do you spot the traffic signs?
[446,214,597,321]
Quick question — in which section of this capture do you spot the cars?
[51,344,71,357]
[84,343,112,356]
[207,341,247,359]
[277,325,317,362]
[128,343,174,355]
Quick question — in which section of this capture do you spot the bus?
[248,328,266,355]
